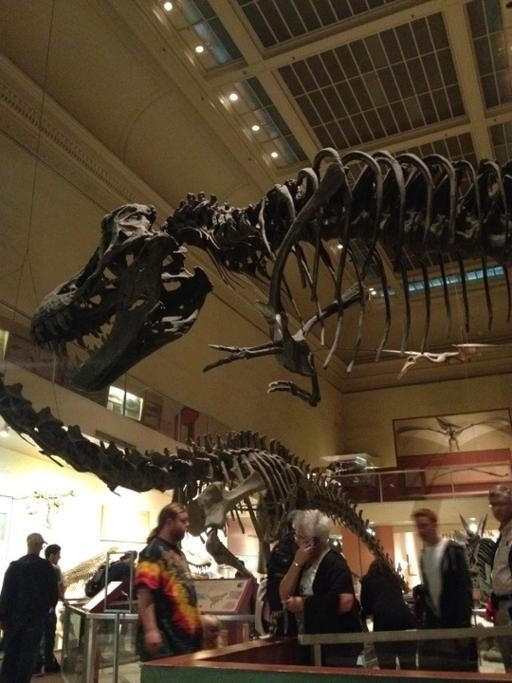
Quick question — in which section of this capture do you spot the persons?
[97,547,137,665]
[276,507,368,668]
[408,584,437,670]
[357,554,420,669]
[264,509,303,637]
[201,610,224,650]
[411,507,480,672]
[131,500,206,662]
[482,483,511,673]
[25,544,69,675]
[1,532,60,682]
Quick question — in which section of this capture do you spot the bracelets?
[291,559,305,570]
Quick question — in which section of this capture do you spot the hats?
[26,532,49,545]
